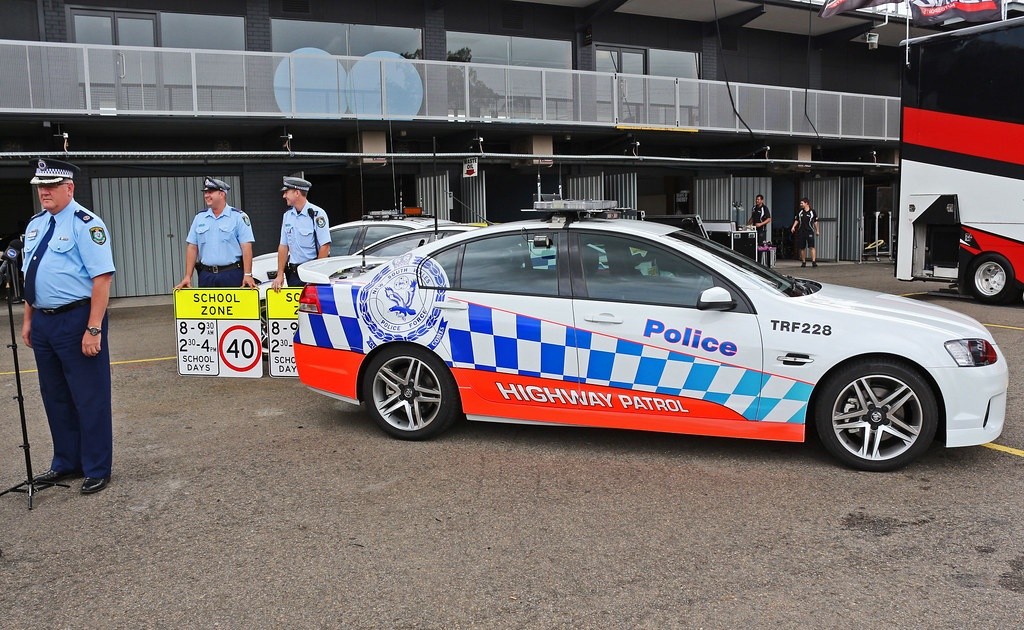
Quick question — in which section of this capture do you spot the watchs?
[87,326,101,336]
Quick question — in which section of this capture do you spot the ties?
[25,217,56,306]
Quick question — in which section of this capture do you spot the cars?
[251,208,486,360]
[293,200,1008,472]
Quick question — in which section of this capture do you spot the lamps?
[870,150,877,163]
[865,32,879,50]
[473,136,483,152]
[763,145,770,159]
[631,141,640,154]
[280,133,293,150]
[54,132,68,151]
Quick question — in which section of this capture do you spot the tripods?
[0,273,70,510]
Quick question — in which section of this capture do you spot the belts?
[39,299,89,315]
[200,263,241,273]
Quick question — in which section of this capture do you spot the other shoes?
[801,262,806,267]
[812,262,818,267]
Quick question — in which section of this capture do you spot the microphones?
[0,239,23,275]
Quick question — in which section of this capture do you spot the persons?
[747,195,771,241]
[175,176,260,290]
[791,197,820,267]
[20,157,116,493]
[271,176,332,294]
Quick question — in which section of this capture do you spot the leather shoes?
[31,469,83,482]
[82,475,112,492]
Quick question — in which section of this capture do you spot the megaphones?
[865,32,879,43]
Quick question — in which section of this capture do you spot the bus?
[894,16,1024,304]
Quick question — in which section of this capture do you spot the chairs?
[580,246,620,286]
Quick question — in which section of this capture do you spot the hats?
[200,176,230,194]
[29,159,80,186]
[280,177,311,192]
